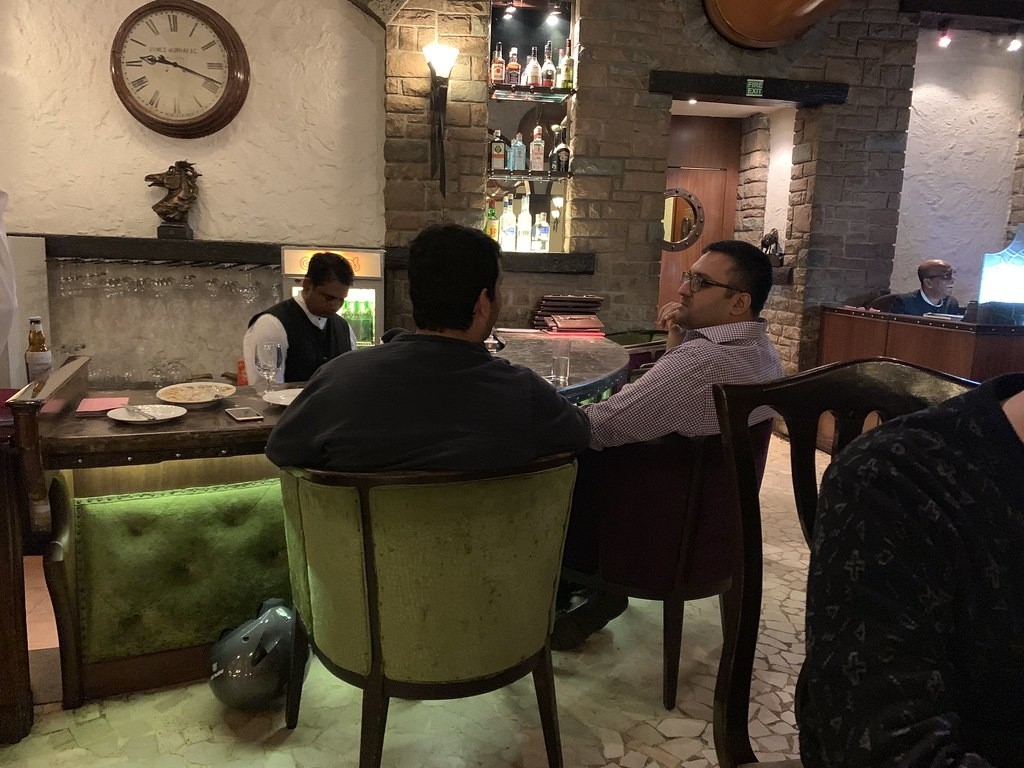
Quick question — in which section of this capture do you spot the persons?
[794,370,1024,768]
[243,253,353,385]
[264,222,591,473]
[551,241,785,650]
[891,259,959,316]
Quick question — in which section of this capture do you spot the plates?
[263,388,304,406]
[107,404,187,425]
[156,382,238,409]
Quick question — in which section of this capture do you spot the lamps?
[422,43,460,198]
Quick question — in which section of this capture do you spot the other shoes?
[550,585,628,651]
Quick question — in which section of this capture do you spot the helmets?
[208,606,311,712]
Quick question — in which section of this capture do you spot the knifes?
[120,403,156,418]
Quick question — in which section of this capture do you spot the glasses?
[924,271,958,280]
[681,271,744,292]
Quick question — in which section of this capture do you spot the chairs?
[606,330,670,348]
[630,363,655,383]
[713,356,984,768]
[279,449,565,768]
[566,417,774,708]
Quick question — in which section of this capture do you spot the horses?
[761,228,779,253]
[145,160,202,223]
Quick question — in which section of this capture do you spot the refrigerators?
[279,245,384,347]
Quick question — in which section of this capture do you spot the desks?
[38,328,672,710]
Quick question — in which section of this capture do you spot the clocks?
[110,0,252,139]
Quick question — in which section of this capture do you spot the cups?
[551,338,572,381]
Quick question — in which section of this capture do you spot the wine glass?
[48,256,291,306]
[51,343,196,389]
[254,342,284,396]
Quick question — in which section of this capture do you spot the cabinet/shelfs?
[488,0,571,252]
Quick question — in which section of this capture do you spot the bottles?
[484,191,551,252]
[490,124,570,174]
[491,39,573,90]
[340,299,374,343]
[25,316,54,384]
